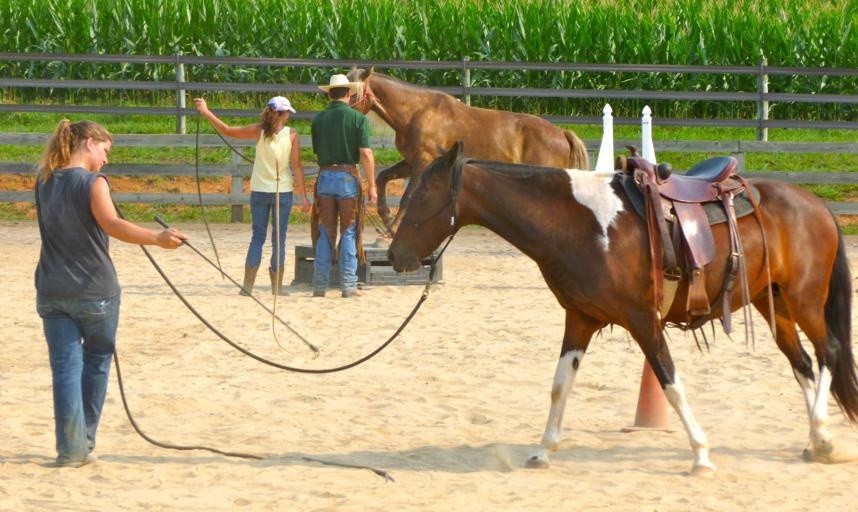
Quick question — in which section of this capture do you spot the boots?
[268,266,286,297]
[236,262,262,297]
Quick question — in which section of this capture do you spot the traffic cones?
[618,356,677,435]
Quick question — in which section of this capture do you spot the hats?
[267,95,297,114]
[317,74,364,94]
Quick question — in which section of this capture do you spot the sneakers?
[311,290,328,298]
[341,288,369,300]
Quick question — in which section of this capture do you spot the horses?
[341,62,589,239]
[384,142,858,479]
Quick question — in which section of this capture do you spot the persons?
[192,96,312,297]
[34,119,187,466]
[312,73,378,297]
